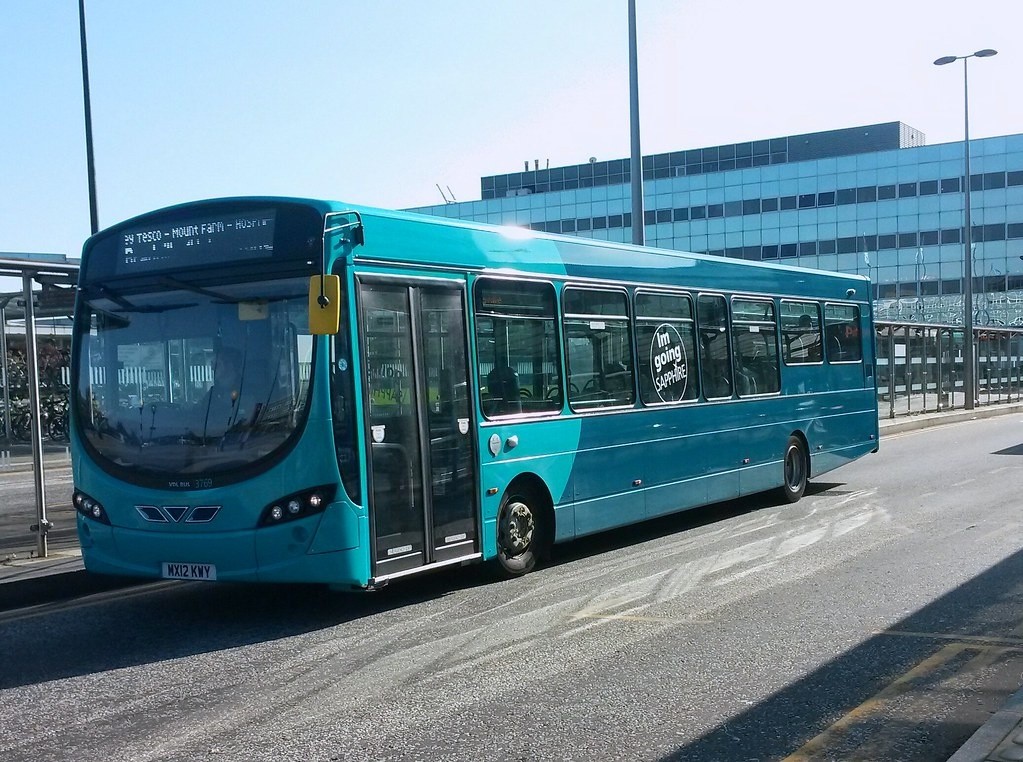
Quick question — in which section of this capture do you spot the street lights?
[934,49,998,409]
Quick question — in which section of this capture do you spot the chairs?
[517,335,844,412]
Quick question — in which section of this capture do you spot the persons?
[197,346,257,411]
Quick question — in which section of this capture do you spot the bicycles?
[0,393,71,444]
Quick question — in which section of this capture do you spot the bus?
[70,197,879,594]
